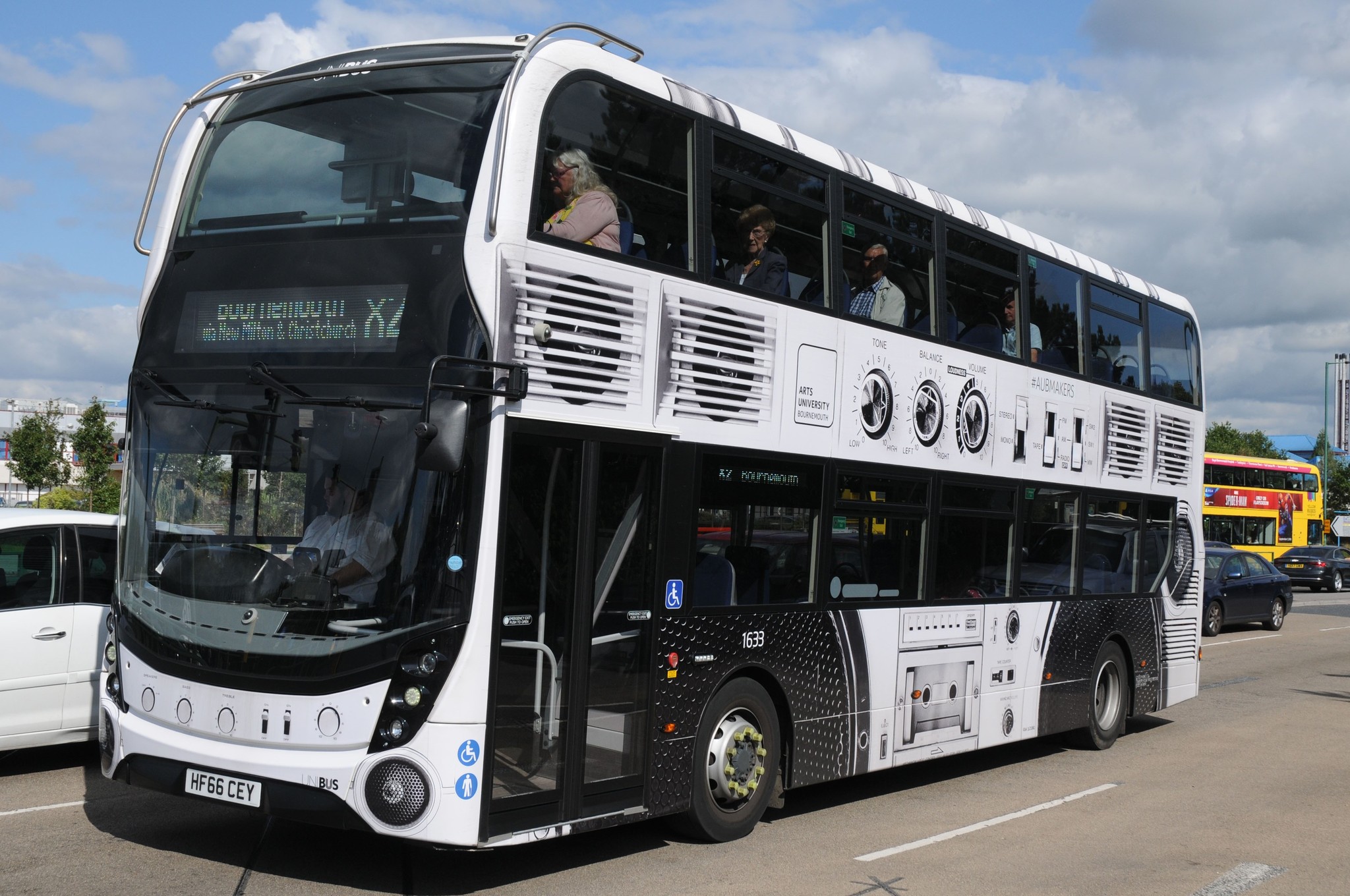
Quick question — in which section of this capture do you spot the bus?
[99,24,1204,855]
[1204,450,1325,563]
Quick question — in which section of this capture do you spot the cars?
[15,501,33,508]
[1202,539,1294,636]
[1274,544,1350,593]
[698,527,869,580]
[754,514,801,526]
[0,506,215,752]
[0,497,8,507]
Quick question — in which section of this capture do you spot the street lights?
[1325,361,1350,543]
[6,398,14,507]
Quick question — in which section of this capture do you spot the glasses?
[547,166,579,180]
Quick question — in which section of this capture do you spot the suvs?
[987,518,1169,597]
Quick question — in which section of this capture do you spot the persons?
[846,243,905,329]
[264,464,398,607]
[1000,291,1043,363]
[1247,535,1260,544]
[541,149,622,253]
[722,205,789,298]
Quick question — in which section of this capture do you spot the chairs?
[616,200,1170,395]
[1205,474,1301,490]
[13,536,51,602]
[692,536,1038,607]
